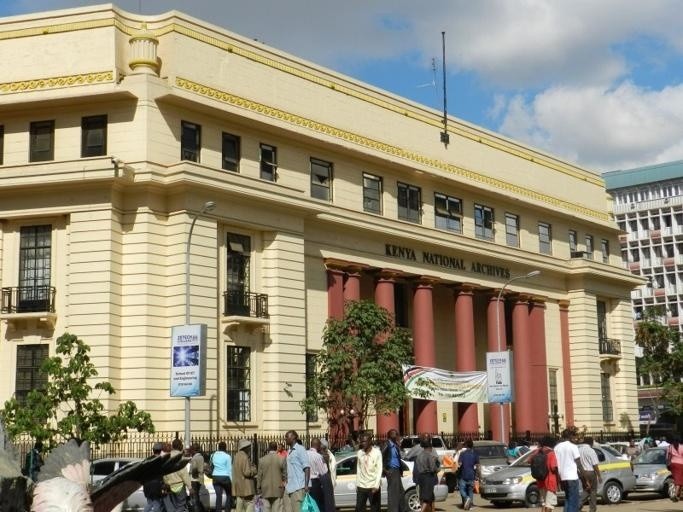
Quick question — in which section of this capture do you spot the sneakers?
[463,495,471,510]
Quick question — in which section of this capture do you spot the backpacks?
[530,446,554,482]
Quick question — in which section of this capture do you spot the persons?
[26,442,44,482]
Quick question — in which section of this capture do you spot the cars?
[87,457,230,510]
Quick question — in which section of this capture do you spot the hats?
[236,437,251,451]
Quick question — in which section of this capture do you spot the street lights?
[496,269,540,443]
[181,199,222,447]
